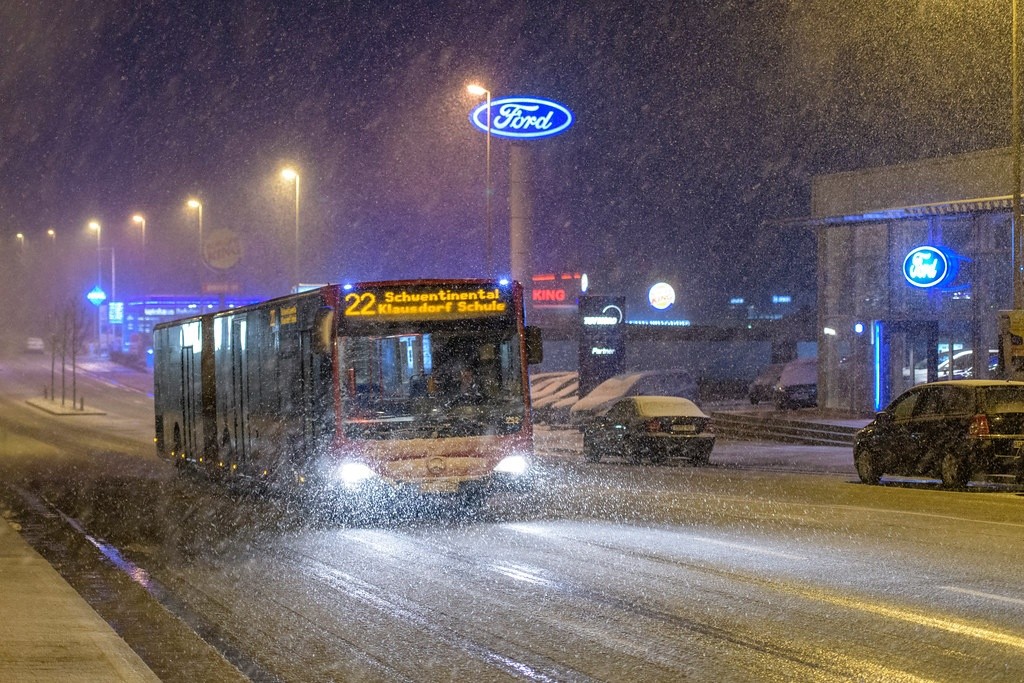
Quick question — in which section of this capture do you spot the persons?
[998,316,1024,371]
[449,366,482,406]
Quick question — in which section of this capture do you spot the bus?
[153,279,543,529]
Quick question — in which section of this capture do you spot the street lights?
[283,171,300,279]
[48,230,56,243]
[468,84,492,280]
[17,233,24,246]
[89,223,101,288]
[132,216,146,251]
[189,201,202,257]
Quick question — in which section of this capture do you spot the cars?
[23,337,45,354]
[773,358,818,409]
[583,396,715,466]
[853,380,1024,491]
[569,370,701,433]
[529,372,578,424]
[748,363,784,404]
